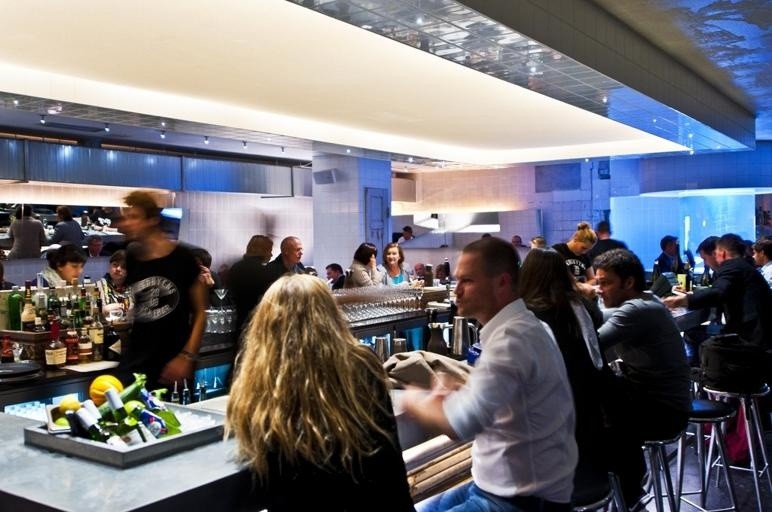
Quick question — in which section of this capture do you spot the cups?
[432,278,440,287]
[109,307,124,320]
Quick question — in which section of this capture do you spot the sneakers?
[630,494,652,511]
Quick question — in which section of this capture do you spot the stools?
[676,399,739,512]
[700,383,772,512]
[642,432,683,512]
[568,469,623,512]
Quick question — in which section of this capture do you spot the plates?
[25,395,237,471]
[0,362,41,378]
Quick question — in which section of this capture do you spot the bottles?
[171,392,179,405]
[96,298,108,325]
[41,319,66,371]
[83,400,106,442]
[81,210,89,227]
[104,388,147,447]
[440,258,451,285]
[76,408,129,449]
[82,300,94,329]
[90,287,99,317]
[182,389,190,406]
[122,292,130,317]
[79,288,88,312]
[83,275,92,303]
[104,326,122,361]
[32,314,46,333]
[65,292,73,318]
[8,286,24,331]
[33,272,47,309]
[20,280,36,331]
[72,294,80,315]
[424,264,433,287]
[48,280,57,299]
[78,328,93,364]
[56,280,68,317]
[53,308,62,330]
[70,278,81,307]
[137,385,181,428]
[73,310,83,328]
[88,307,104,362]
[198,386,206,402]
[47,286,61,317]
[130,404,182,439]
[42,217,48,229]
[64,315,79,365]
[35,294,50,331]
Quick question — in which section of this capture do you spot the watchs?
[179,350,199,364]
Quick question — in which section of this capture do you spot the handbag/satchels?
[697,334,767,392]
[705,399,748,462]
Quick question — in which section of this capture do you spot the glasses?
[390,244,399,246]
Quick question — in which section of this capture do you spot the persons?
[215,263,231,279]
[325,264,344,288]
[587,220,630,265]
[662,233,772,382]
[91,206,122,227]
[103,250,126,290]
[435,264,451,282]
[221,273,415,512]
[696,235,747,272]
[30,243,87,288]
[742,240,760,269]
[116,190,207,406]
[194,247,234,312]
[654,235,691,281]
[352,244,382,287]
[0,264,15,289]
[265,237,319,278]
[520,247,617,480]
[751,238,772,290]
[378,242,410,286]
[396,225,415,244]
[88,236,107,255]
[553,221,605,330]
[414,263,432,287]
[402,240,579,512]
[41,205,85,256]
[5,205,49,258]
[224,235,274,358]
[594,249,692,512]
[513,236,547,248]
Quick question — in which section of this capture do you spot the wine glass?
[214,289,228,312]
[204,309,233,334]
[332,283,424,324]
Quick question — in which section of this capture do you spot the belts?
[512,497,572,511]
